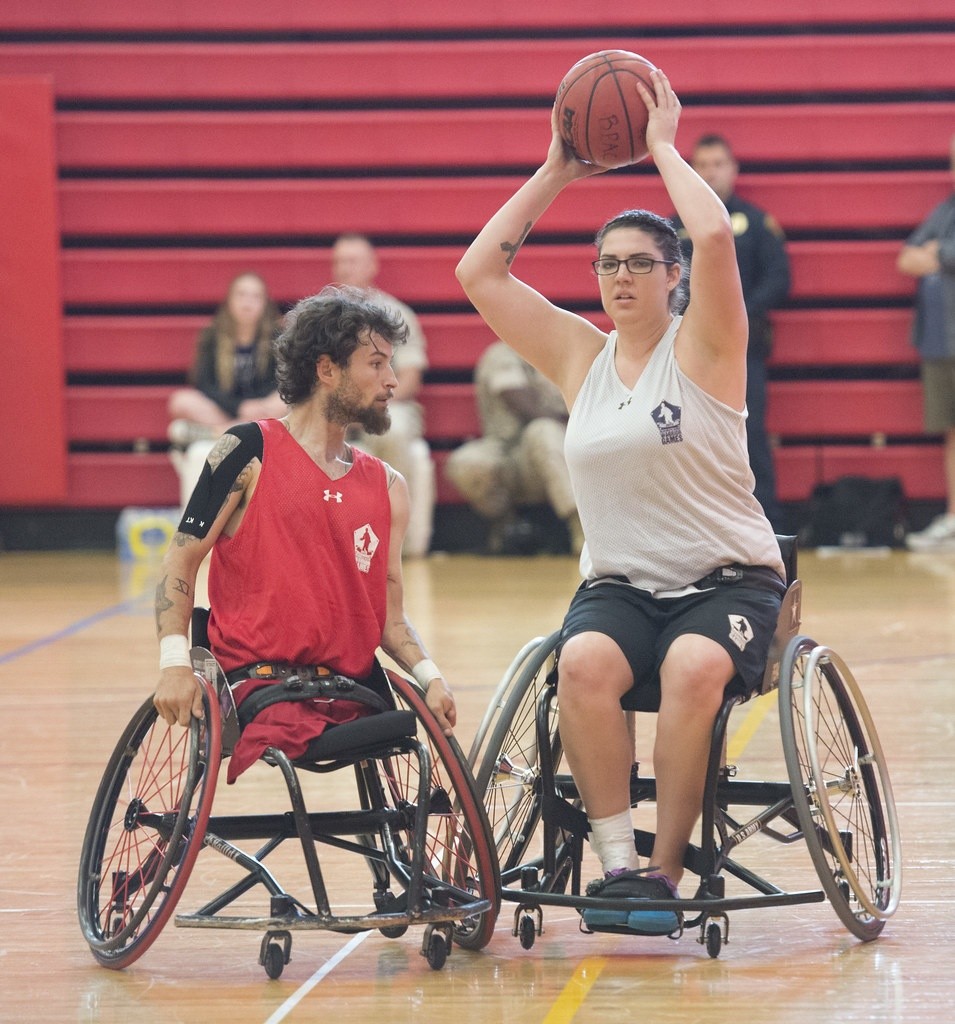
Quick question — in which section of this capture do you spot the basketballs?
[553,48,661,168]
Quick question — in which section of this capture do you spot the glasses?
[592,258,674,275]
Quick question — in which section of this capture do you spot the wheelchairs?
[73,598,503,981]
[435,528,904,949]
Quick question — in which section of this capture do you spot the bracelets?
[155,634,193,669]
[410,658,442,691]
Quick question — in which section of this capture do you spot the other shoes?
[626,873,683,932]
[901,514,955,550]
[584,868,629,925]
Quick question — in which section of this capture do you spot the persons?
[162,271,282,524]
[454,69,788,937]
[150,281,454,785]
[894,194,955,552]
[329,234,437,559]
[442,337,584,558]
[668,131,798,600]
